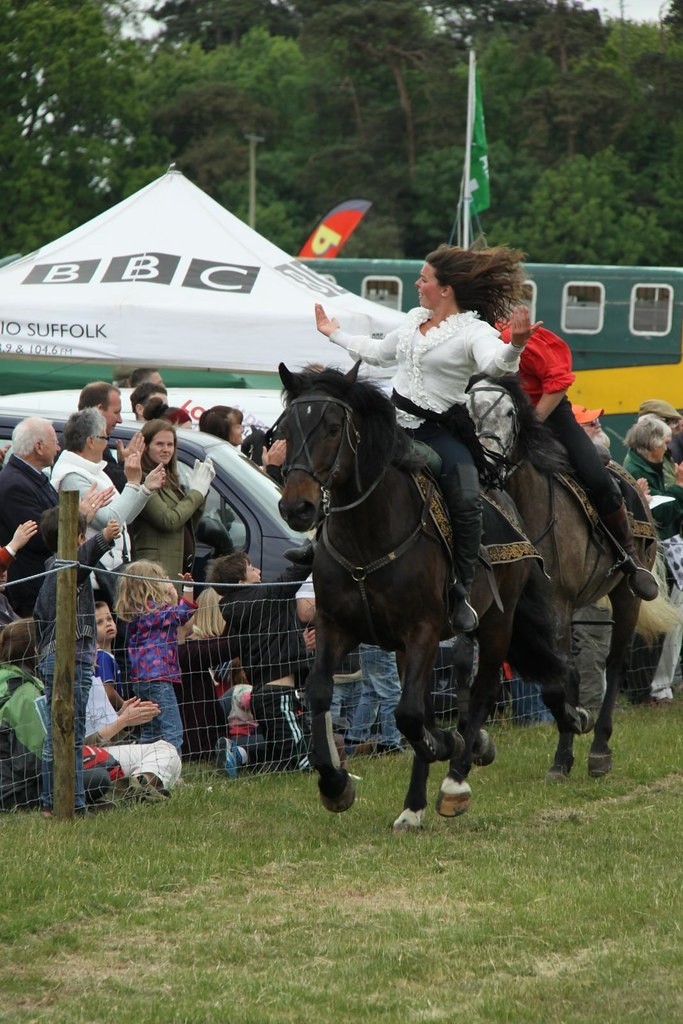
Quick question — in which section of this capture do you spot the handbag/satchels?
[658,533,683,590]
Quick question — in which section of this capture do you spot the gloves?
[185,453,216,498]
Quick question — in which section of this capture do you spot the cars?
[1,408,314,588]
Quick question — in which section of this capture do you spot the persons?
[0,365,683,818]
[493,317,658,601]
[315,232,544,632]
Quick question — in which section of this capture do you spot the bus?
[294,256,683,489]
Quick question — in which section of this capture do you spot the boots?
[599,500,658,601]
[442,461,484,632]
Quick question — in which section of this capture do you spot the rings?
[91,503,95,508]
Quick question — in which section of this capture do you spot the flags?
[469,72,493,223]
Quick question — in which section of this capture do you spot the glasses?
[580,418,600,428]
[89,435,109,443]
[42,440,59,449]
[659,417,679,428]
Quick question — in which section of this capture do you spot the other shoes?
[43,806,53,817]
[343,738,374,755]
[86,797,117,811]
[130,774,167,802]
[215,736,235,775]
[376,743,407,754]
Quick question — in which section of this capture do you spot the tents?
[0,163,411,396]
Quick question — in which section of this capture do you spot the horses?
[276,357,659,835]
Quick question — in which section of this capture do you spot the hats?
[571,404,604,425]
[638,400,682,420]
